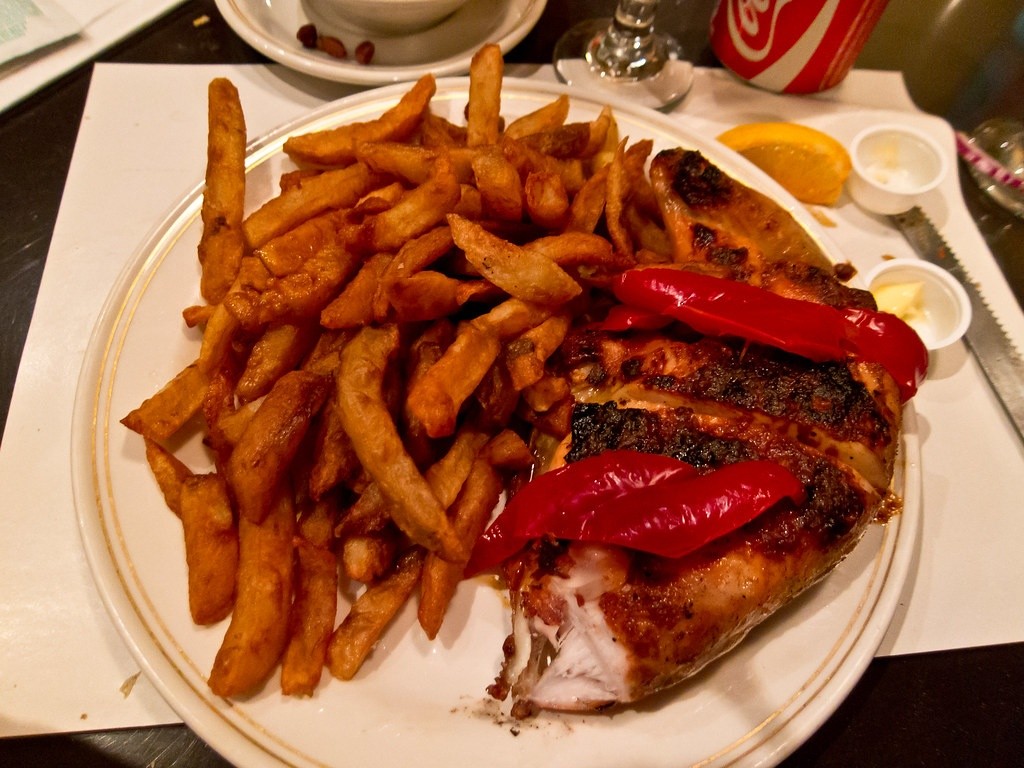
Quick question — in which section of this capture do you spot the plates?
[68,76,924,768]
[212,0,548,87]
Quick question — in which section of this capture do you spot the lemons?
[714,122,855,207]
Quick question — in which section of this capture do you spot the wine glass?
[552,0,695,109]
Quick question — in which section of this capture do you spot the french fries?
[115,40,653,700]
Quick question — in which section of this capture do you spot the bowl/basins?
[864,257,973,351]
[844,124,948,216]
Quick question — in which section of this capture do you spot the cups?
[329,0,466,36]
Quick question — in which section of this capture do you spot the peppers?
[602,264,931,400]
[466,451,804,561]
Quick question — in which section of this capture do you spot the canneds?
[708,0,890,93]
